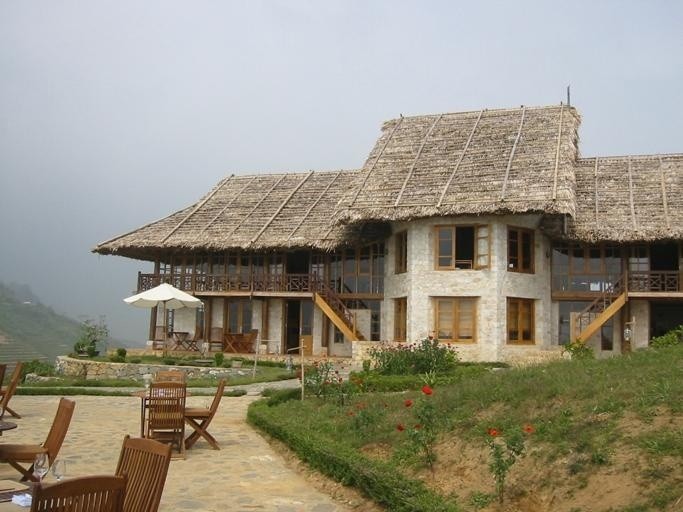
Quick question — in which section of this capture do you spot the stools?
[185,339,199,351]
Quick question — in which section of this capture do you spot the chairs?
[150,325,168,350]
[29,472,128,512]
[0,362,23,421]
[238,329,257,353]
[146,370,226,460]
[0,396,75,483]
[0,364,7,394]
[113,435,173,512]
[207,326,225,352]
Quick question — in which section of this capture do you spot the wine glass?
[33,453,49,482]
[52,458,66,482]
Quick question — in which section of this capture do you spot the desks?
[168,331,188,351]
[0,422,17,438]
[222,331,242,353]
[131,390,190,438]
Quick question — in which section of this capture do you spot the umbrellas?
[121,281,203,347]
